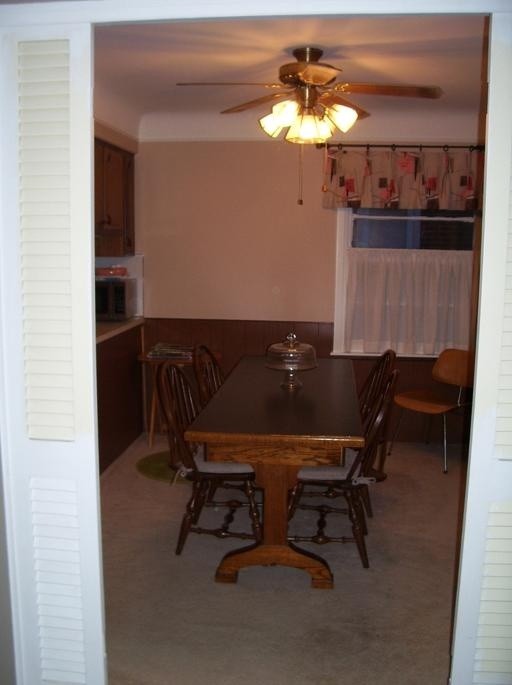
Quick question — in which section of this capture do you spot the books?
[147,343,193,361]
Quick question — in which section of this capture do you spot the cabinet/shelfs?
[95,326,144,475]
[94,136,135,257]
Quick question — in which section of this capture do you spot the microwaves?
[94,276,136,322]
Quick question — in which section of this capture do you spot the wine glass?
[265,333,319,392]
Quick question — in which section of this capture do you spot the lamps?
[259,87,360,146]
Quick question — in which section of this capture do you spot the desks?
[138,351,192,450]
[184,354,365,590]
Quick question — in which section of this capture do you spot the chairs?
[387,348,469,474]
[156,361,267,567]
[358,349,396,413]
[193,343,225,405]
[270,368,400,569]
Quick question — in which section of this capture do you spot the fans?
[176,45,444,119]
